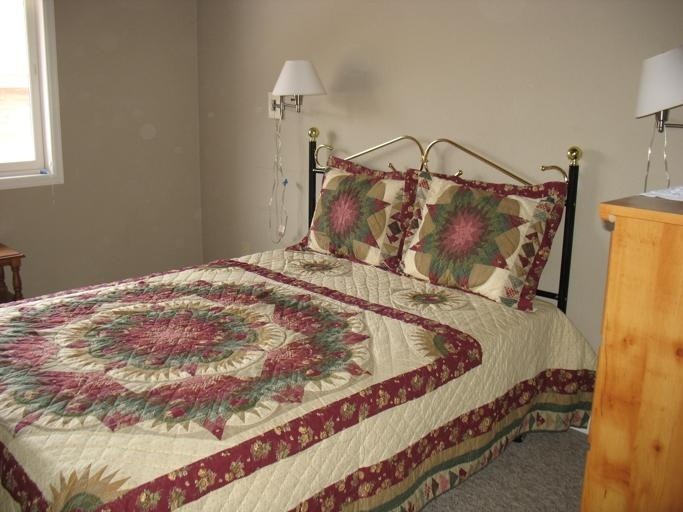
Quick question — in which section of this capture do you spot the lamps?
[268,60,325,121]
[634,48,683,133]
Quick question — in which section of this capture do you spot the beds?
[0,127,597,512]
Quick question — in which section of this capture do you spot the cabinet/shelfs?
[581,189,683,511]
